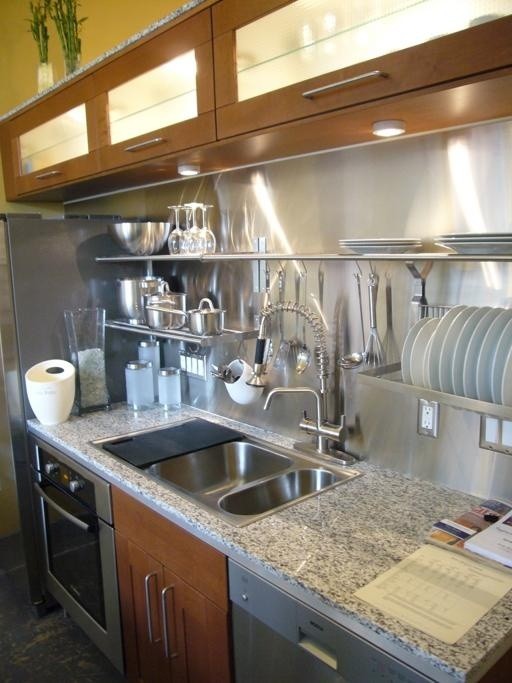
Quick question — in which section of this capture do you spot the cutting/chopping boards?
[101,418,248,469]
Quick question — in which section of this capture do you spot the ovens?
[27,433,124,683]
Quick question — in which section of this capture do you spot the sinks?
[218,468,366,529]
[145,442,294,496]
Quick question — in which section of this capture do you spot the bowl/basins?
[110,221,171,255]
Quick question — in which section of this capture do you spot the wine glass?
[167,201,217,256]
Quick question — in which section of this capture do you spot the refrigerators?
[228,558,455,682]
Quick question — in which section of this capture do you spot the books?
[426,498,512,572]
[464,506,512,567]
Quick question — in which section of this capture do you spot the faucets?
[263,387,324,423]
[246,301,328,387]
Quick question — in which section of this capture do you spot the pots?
[115,273,225,336]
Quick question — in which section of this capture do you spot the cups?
[62,306,111,416]
[224,360,265,405]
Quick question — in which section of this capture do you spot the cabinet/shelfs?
[109,484,234,683]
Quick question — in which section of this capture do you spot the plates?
[401,303,511,406]
[432,232,512,252]
[339,238,425,251]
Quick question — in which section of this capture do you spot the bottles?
[125,339,182,412]
